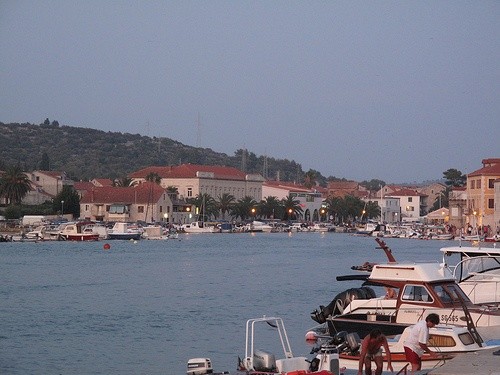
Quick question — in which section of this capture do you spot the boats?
[186,246,500,375]
[0,216,500,242]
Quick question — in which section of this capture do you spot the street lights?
[363,210,366,224]
[252,208,255,222]
[442,211,444,223]
[61,200,64,216]
[163,213,168,228]
[288,209,292,225]
[322,209,325,221]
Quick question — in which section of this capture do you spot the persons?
[466,223,473,234]
[384,286,397,300]
[358,328,393,375]
[404,314,439,372]
